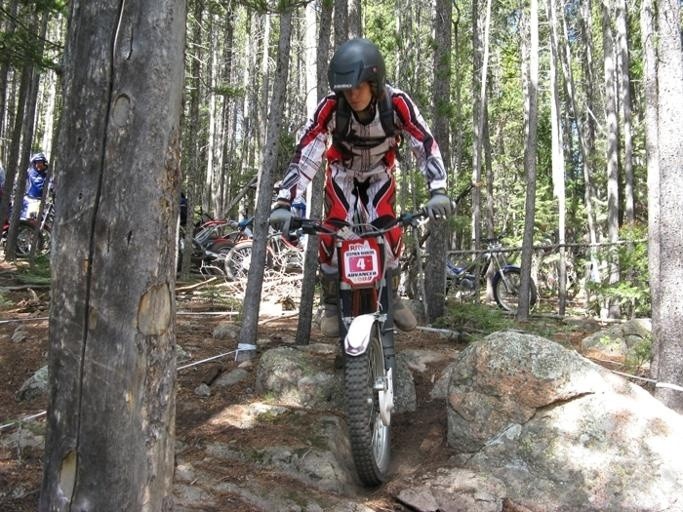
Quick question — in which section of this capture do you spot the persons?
[272,177,306,245]
[270,39,455,339]
[25,152,49,199]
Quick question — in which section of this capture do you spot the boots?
[319,268,341,337]
[384,264,418,332]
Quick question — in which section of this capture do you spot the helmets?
[32,153,47,163]
[327,38,386,100]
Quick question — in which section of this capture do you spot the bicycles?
[0,183,56,259]
[178,215,314,289]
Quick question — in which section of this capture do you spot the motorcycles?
[263,200,459,488]
[403,233,536,314]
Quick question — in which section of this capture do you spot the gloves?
[269,207,292,238]
[425,193,451,225]
